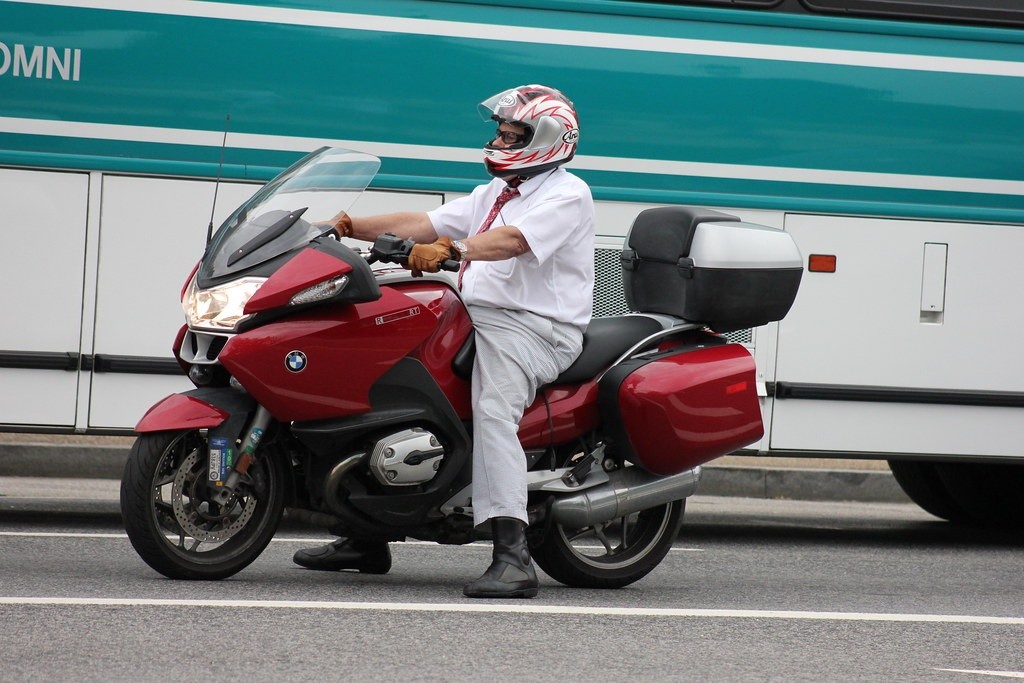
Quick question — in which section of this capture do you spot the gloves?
[312,210,356,238]
[408,236,452,273]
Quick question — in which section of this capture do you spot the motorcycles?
[118,140,804,583]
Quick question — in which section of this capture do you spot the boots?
[463,518,538,598]
[293,537,392,575]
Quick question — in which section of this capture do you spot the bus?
[1,1,1023,524]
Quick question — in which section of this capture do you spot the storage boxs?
[618,204,802,333]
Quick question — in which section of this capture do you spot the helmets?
[482,84,580,178]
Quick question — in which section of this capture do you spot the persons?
[294,84,595,598]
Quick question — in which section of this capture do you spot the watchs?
[452,240,468,261]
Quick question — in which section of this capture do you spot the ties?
[458,186,521,293]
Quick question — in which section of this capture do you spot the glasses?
[496,129,525,144]
